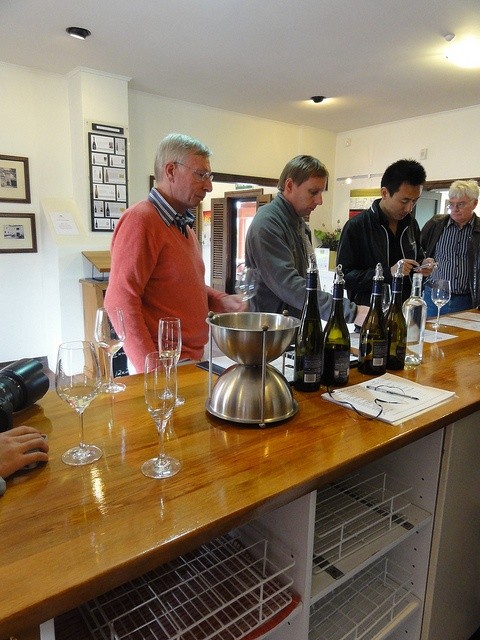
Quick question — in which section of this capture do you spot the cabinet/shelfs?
[39,406,479,639]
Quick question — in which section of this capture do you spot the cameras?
[0,358,49,433]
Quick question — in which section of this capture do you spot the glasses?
[447,198,474,208]
[175,162,214,182]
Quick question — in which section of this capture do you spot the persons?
[243,153,377,351]
[336,160,436,322]
[103,132,248,376]
[417,179,480,319]
[0,425,50,496]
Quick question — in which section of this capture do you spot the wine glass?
[427,329,446,361]
[55,341,102,466]
[141,352,181,479]
[431,279,451,328]
[234,267,261,301]
[159,317,182,401]
[93,307,126,395]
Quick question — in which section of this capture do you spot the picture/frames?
[88,133,128,233]
[0,213,37,253]
[93,124,124,134]
[0,155,31,204]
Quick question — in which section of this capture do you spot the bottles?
[358,263,388,377]
[295,253,322,393]
[402,273,428,366]
[385,259,407,372]
[322,264,351,388]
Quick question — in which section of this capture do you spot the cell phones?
[414,262,438,271]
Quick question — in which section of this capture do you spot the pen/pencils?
[367,385,419,400]
